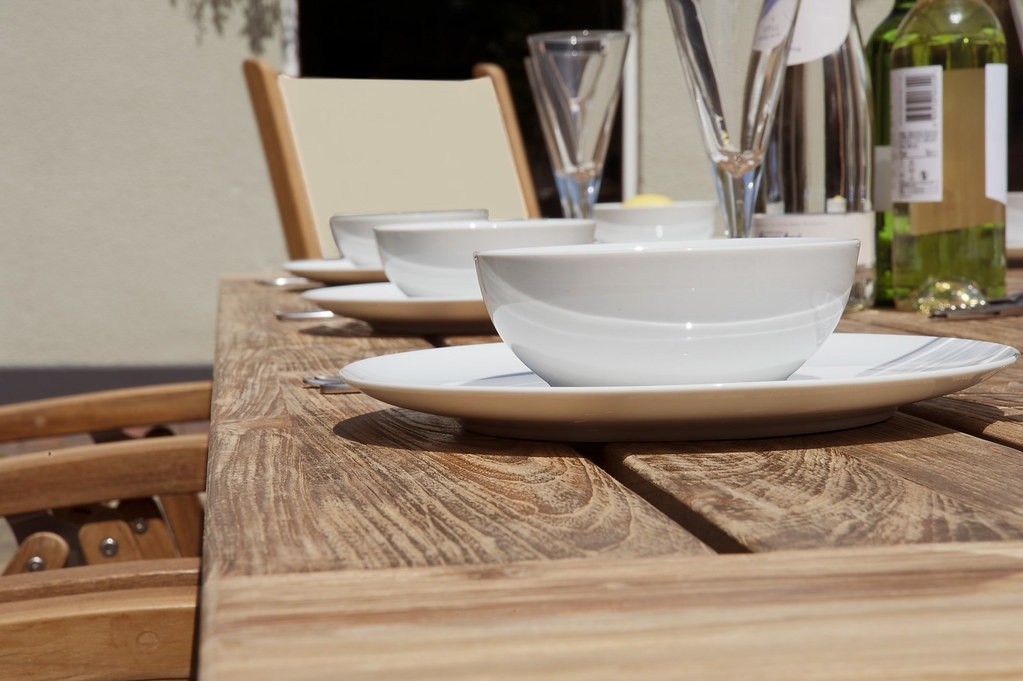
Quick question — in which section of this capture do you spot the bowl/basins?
[330,210,492,264]
[374,216,598,301]
[477,232,862,389]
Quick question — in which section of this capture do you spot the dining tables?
[197,263,1023,681]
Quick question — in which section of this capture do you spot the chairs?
[242,55,539,262]
[2,382,214,681]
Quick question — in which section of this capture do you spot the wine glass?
[521,26,631,221]
[661,1,799,239]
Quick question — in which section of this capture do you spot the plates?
[343,330,1021,441]
[303,282,506,333]
[285,254,391,282]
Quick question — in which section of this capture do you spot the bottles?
[892,0,1009,315]
[864,0,896,306]
